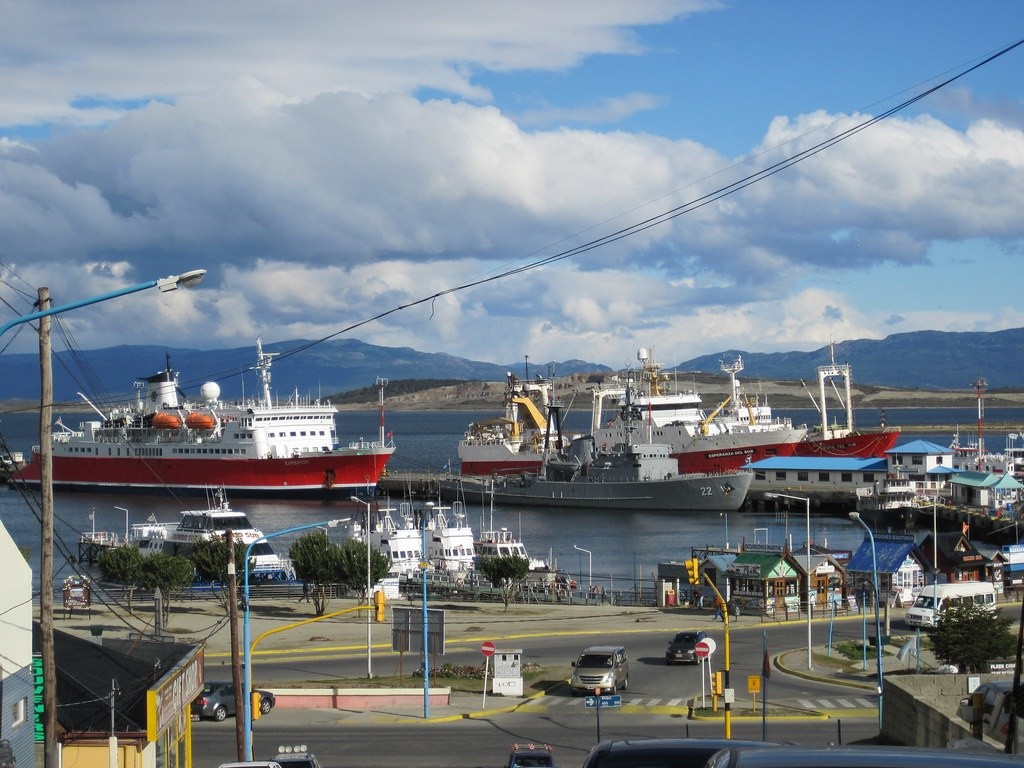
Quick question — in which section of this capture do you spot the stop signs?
[695,642,710,658]
[480,642,496,657]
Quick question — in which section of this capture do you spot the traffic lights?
[684,558,699,585]
[710,671,720,695]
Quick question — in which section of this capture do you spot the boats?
[438,372,755,513]
[855,478,921,532]
[129,486,576,591]
[574,344,903,474]
[7,335,396,500]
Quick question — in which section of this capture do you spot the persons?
[299,583,310,603]
[714,595,724,620]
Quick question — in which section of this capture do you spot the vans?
[572,645,628,695]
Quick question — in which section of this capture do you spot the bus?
[905,584,996,629]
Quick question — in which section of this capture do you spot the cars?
[956,680,1024,753]
[666,629,708,664]
[191,680,274,721]
[218,740,1023,767]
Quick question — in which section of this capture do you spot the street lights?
[848,513,884,699]
[573,546,592,599]
[924,496,938,628]
[244,498,433,763]
[765,492,812,670]
